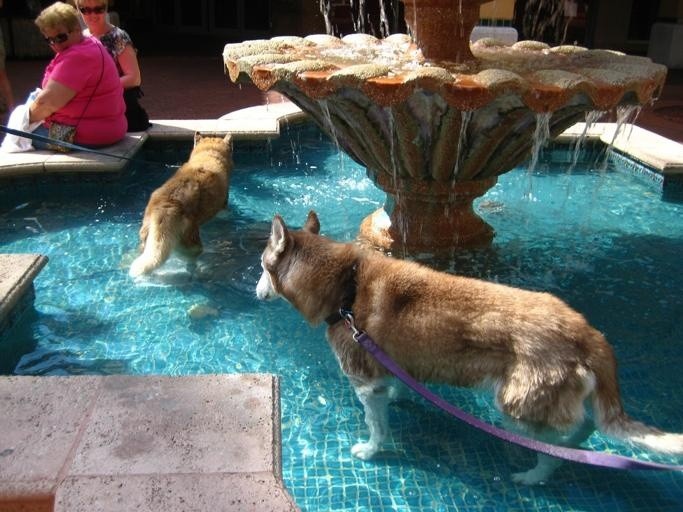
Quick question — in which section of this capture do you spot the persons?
[74,0,152,132]
[5,1,129,151]
[0,30,15,115]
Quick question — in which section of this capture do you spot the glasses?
[78,5,106,17]
[44,30,71,47]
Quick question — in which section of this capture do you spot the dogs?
[127,131,236,278]
[251,208,683,487]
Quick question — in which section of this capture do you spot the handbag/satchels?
[47,122,76,153]
